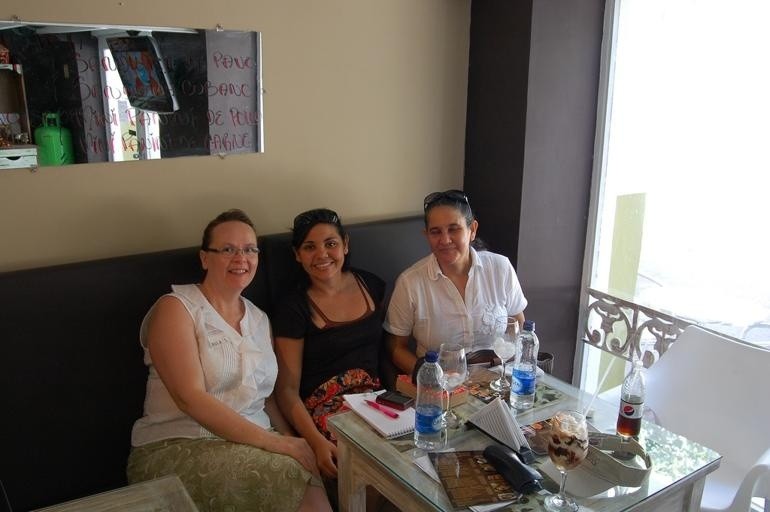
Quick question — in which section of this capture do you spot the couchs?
[0,215,433,512]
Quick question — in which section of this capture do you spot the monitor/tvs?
[107,35,179,114]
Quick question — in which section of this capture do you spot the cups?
[511,320,541,410]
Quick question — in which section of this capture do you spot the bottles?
[415,351,443,450]
[617,358,646,436]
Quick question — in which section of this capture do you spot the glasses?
[424,189,471,212]
[293,208,341,229]
[205,245,259,257]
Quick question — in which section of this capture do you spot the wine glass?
[545,410,588,512]
[438,344,469,425]
[490,314,520,394]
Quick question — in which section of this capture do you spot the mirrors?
[0,20,265,170]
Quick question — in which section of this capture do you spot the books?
[341,388,417,438]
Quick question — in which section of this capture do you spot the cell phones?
[377,390,415,410]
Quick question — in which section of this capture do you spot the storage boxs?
[0,149,38,169]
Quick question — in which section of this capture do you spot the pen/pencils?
[364,399,399,419]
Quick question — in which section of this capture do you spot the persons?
[382,189,529,376]
[275,210,387,480]
[128,205,333,512]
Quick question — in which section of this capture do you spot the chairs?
[597,325,770,512]
